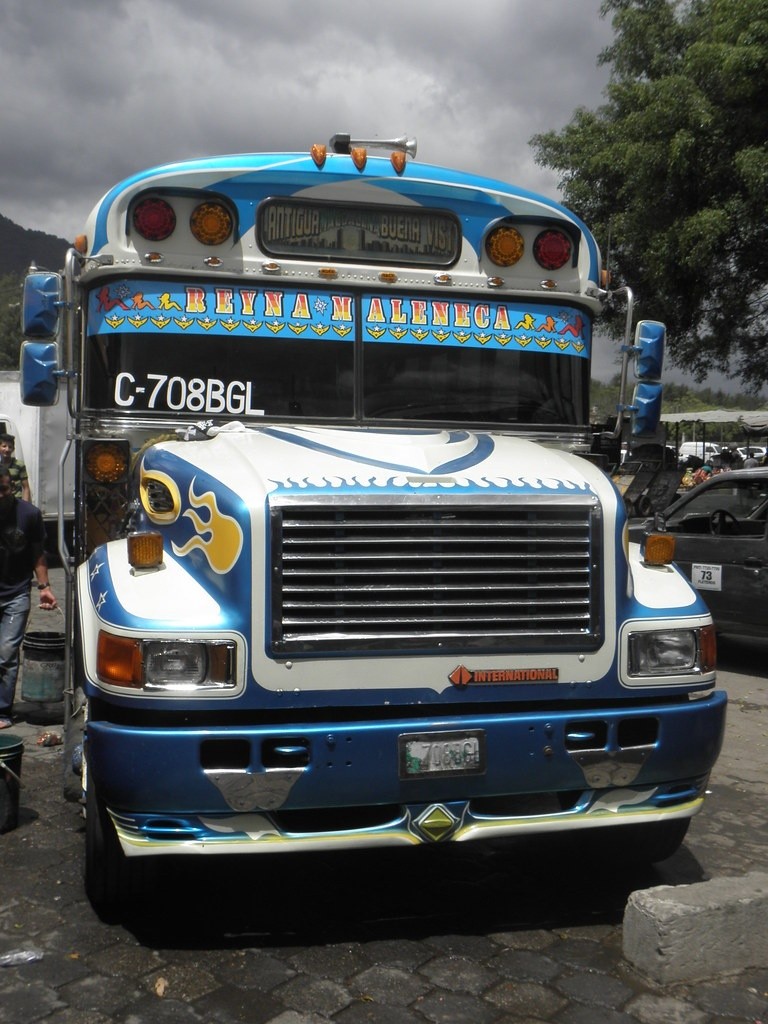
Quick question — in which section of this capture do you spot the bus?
[18,133,731,902]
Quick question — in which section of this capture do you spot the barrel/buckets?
[22,604,66,702]
[0,732,25,835]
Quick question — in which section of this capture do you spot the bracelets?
[38,582,50,589]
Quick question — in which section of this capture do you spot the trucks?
[0,367,77,560]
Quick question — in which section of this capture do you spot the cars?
[625,467,768,640]
[620,440,768,469]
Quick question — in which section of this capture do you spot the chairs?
[130,338,229,411]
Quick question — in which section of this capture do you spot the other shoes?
[0,717,11,728]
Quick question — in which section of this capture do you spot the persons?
[744,452,759,468]
[0,433,31,503]
[0,463,57,727]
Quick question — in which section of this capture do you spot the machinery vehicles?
[590,414,688,520]
